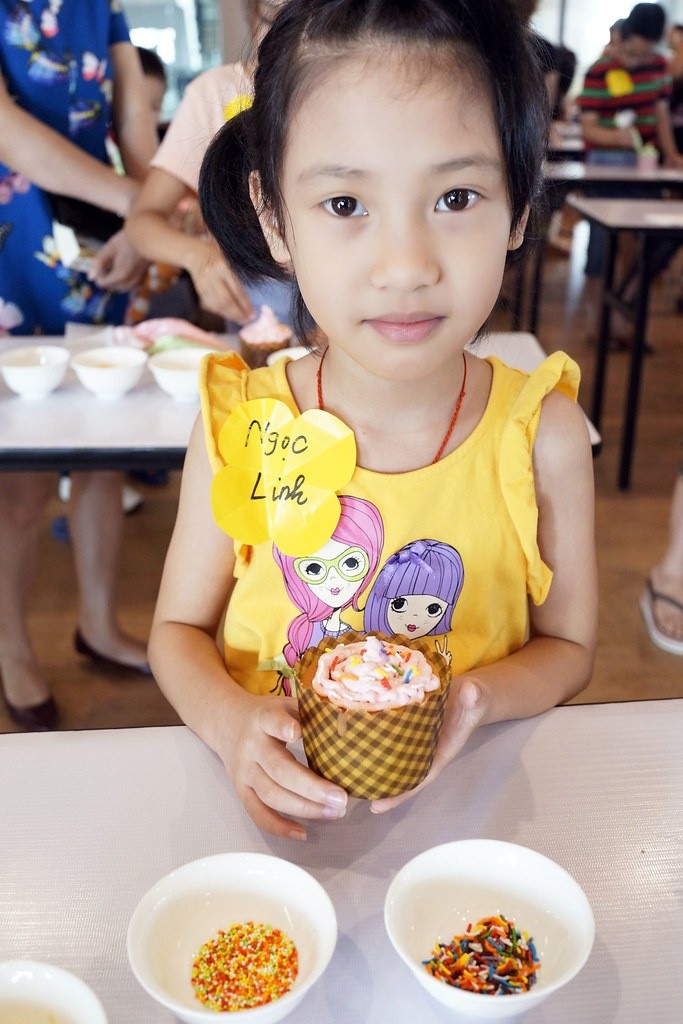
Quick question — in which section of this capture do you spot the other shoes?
[585,327,653,356]
[0,668,62,731]
[73,622,154,680]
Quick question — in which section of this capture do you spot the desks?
[0,695,683,1024]
[566,197,683,495]
[507,162,683,336]
[0,334,303,470]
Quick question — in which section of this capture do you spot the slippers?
[633,574,683,656]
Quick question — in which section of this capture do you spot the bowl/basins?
[148,347,222,402]
[0,958,108,1024]
[70,346,148,401]
[265,346,320,368]
[384,839,595,1019]
[0,345,72,398]
[127,852,338,1024]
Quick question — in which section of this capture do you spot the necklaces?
[317,345,467,463]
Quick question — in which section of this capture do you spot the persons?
[0,0,683,734]
[144,0,600,841]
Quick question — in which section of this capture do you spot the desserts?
[313,638,438,714]
[239,306,292,367]
